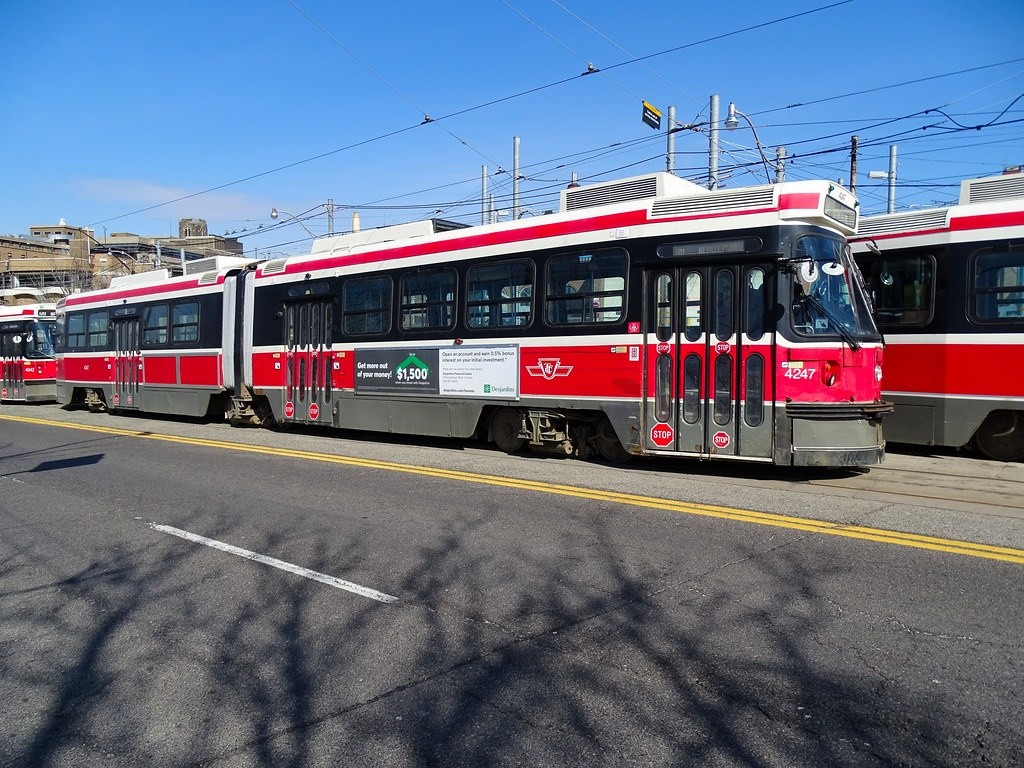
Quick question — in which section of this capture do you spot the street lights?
[868,145,897,214]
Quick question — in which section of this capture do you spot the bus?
[847,164,1024,465]
[0,301,59,404]
[54,171,896,471]
